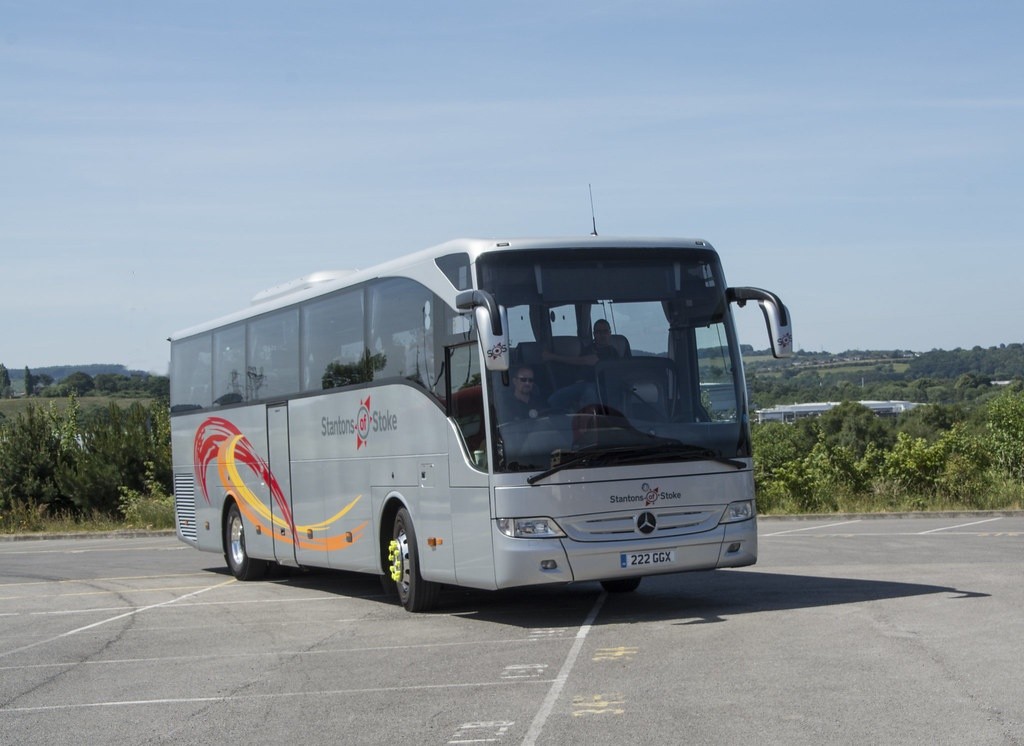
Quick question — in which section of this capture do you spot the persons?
[580,320,617,360]
[511,365,539,419]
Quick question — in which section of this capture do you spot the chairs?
[215,337,681,438]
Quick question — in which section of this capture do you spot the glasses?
[593,329,610,334]
[516,376,534,382]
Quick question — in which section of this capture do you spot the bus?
[166,238,796,613]
